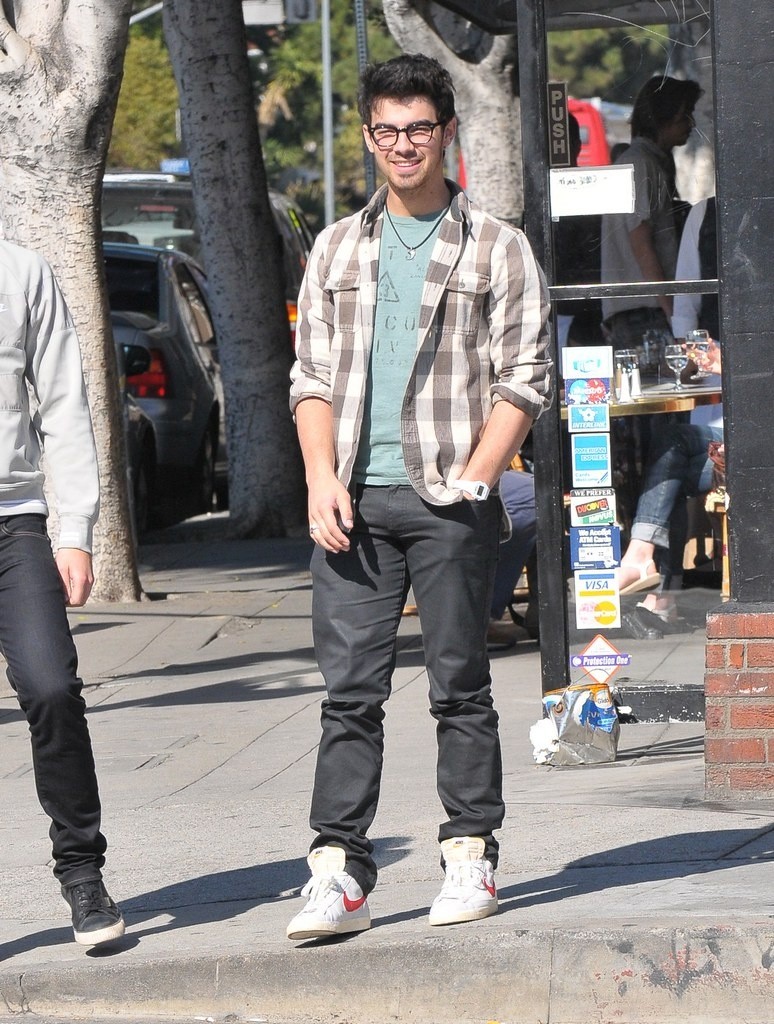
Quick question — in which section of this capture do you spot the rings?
[310,524,317,530]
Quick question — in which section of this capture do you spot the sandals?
[619,559,660,596]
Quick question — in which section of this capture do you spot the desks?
[528,363,721,551]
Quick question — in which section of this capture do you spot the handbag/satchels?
[653,200,692,281]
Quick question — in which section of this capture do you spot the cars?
[102,241,232,528]
[112,339,162,545]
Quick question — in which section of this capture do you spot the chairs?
[699,484,730,604]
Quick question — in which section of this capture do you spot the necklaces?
[384,198,452,260]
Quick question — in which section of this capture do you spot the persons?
[485,467,570,653]
[555,74,726,598]
[284,51,556,943]
[0,236,127,948]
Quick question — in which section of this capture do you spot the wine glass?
[686,329,711,380]
[644,334,666,391]
[647,328,673,394]
[665,345,688,391]
[614,348,642,405]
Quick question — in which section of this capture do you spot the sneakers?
[286,846,371,940]
[60,880,125,946]
[428,836,498,926]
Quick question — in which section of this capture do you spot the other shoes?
[485,622,516,651]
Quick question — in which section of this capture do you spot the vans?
[100,169,319,353]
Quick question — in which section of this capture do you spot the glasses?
[368,119,443,147]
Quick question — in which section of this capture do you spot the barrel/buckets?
[542,669,620,766]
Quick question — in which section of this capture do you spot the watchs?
[452,480,490,502]
